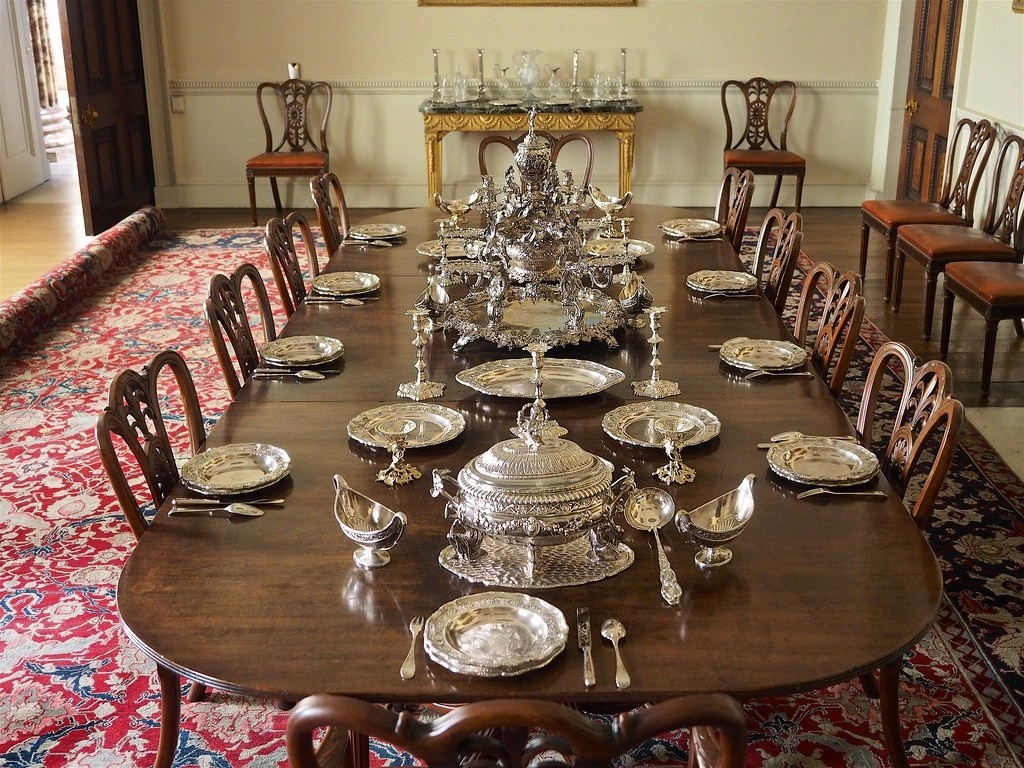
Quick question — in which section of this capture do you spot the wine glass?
[432,189,481,223]
[588,183,633,237]
[332,473,407,567]
[676,473,757,568]
[618,271,654,328]
[413,275,450,332]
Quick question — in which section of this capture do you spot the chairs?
[851,343,965,696]
[262,212,319,321]
[309,171,350,261]
[938,256,1024,400]
[751,202,803,321]
[96,349,209,704]
[205,264,278,406]
[721,75,808,227]
[713,168,754,250]
[286,692,750,768]
[891,135,1024,343]
[244,78,333,228]
[858,118,997,303]
[794,262,866,400]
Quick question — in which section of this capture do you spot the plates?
[662,219,722,238]
[348,224,407,239]
[455,357,625,399]
[311,271,380,296]
[587,239,656,256]
[600,400,721,448]
[181,443,291,495]
[719,340,807,371]
[686,270,757,293]
[416,238,486,260]
[347,402,466,447]
[424,591,569,677]
[258,335,343,366]
[766,437,880,487]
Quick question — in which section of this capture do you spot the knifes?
[576,608,595,687]
[172,498,285,504]
[254,369,341,373]
[304,297,379,300]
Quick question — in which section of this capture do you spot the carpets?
[0,206,1024,768]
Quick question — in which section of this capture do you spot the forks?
[676,236,725,243]
[342,240,393,247]
[745,371,815,380]
[796,488,888,499]
[401,617,424,678]
[703,294,762,299]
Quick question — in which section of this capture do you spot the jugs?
[440,50,610,102]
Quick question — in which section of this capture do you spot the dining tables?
[116,209,942,768]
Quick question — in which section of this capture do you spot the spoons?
[770,431,856,442]
[252,370,325,379]
[722,336,791,345]
[305,298,364,306]
[601,618,632,688]
[168,503,264,516]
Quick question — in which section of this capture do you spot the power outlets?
[171,96,185,114]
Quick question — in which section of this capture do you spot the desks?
[418,97,643,209]
